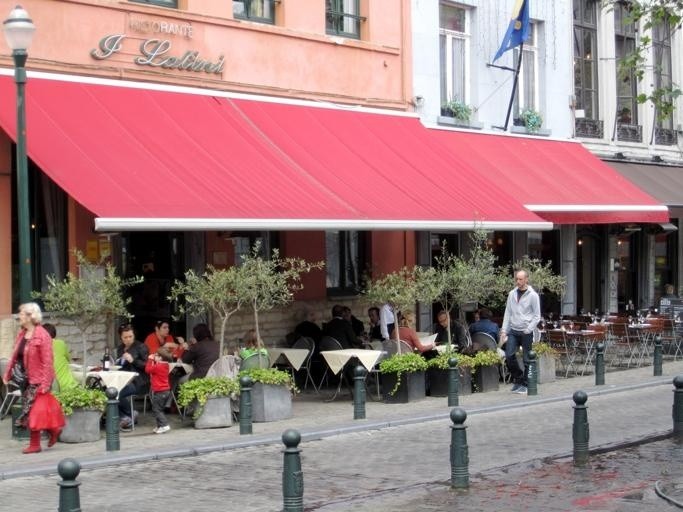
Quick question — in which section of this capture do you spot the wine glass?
[549,313,553,325]
[601,315,607,325]
[558,313,564,324]
[628,315,633,328]
[581,308,585,317]
[552,321,560,332]
[637,310,641,323]
[595,309,599,319]
[640,317,646,327]
[591,315,595,326]
[71,351,82,365]
[569,323,576,334]
[540,321,547,332]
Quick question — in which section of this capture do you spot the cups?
[172,353,177,363]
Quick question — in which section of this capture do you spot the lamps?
[618,241,622,245]
[578,240,584,247]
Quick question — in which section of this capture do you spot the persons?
[177,324,218,415]
[41,323,73,392]
[116,324,150,428]
[500,269,540,393]
[368,307,385,341]
[323,305,365,350]
[390,310,436,353]
[144,321,185,359]
[469,309,498,342]
[434,310,466,354]
[380,302,395,341]
[3,303,65,453]
[144,349,171,434]
[657,283,677,307]
[344,306,367,341]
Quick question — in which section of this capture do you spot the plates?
[69,365,84,372]
[109,365,122,371]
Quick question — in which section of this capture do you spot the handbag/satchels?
[29,387,67,432]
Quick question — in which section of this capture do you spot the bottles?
[102,346,109,371]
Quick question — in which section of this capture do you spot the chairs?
[534,327,541,343]
[240,352,271,372]
[183,355,242,423]
[0,358,22,420]
[316,336,353,391]
[289,336,319,396]
[642,318,663,366]
[657,316,683,361]
[650,315,664,319]
[546,331,578,379]
[471,332,511,384]
[581,323,607,372]
[387,339,414,358]
[126,393,149,431]
[565,325,580,331]
[610,319,637,368]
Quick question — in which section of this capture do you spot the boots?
[22,430,42,454]
[47,426,62,448]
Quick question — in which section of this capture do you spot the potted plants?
[177,376,238,429]
[427,352,475,396]
[50,383,109,443]
[513,109,543,134]
[515,342,558,384]
[440,95,471,121]
[236,367,296,423]
[473,350,503,393]
[379,352,429,403]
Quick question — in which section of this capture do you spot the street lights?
[2,4,36,439]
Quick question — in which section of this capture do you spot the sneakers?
[511,382,523,392]
[156,425,170,434]
[152,428,158,433]
[517,386,528,395]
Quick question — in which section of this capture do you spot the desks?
[566,330,604,376]
[414,344,459,355]
[169,362,193,377]
[319,349,389,402]
[73,366,140,400]
[628,324,659,368]
[270,348,309,371]
